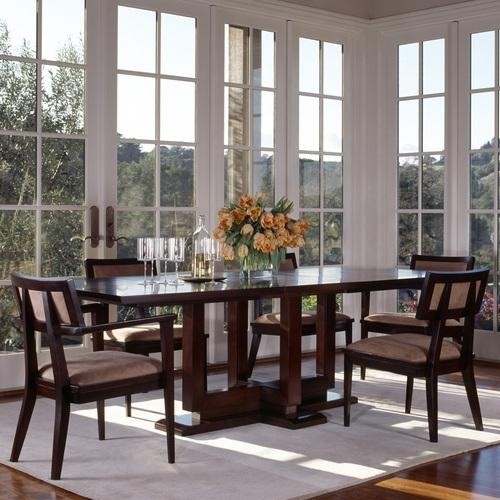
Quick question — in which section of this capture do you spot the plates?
[177,275,227,283]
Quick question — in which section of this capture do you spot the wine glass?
[135,237,184,286]
[203,239,222,285]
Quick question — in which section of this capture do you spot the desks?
[74,265,428,437]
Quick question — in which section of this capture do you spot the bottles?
[192,214,212,276]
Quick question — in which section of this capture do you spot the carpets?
[0,371,497,498]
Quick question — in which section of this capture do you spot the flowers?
[211,189,312,272]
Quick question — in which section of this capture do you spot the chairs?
[344,266,488,443]
[9,271,177,481]
[246,254,490,412]
[84,258,210,417]
